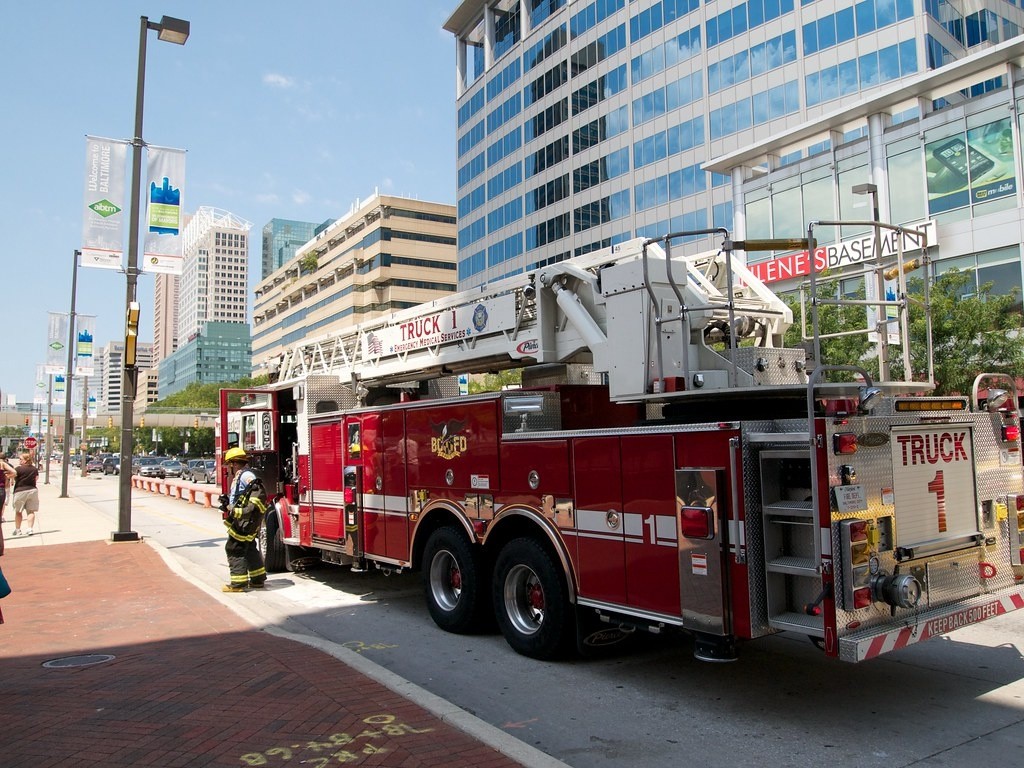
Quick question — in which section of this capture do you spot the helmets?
[224,446,249,465]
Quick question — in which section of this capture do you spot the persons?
[218,447,267,592]
[11,452,40,536]
[0,459,16,555]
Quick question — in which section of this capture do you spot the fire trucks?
[213,218,1024,671]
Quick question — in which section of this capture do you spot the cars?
[190,459,215,484]
[50,453,199,480]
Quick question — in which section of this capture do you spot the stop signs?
[23,437,37,449]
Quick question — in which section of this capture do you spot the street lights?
[851,183,880,223]
[109,13,191,543]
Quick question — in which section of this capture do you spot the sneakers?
[26,528,34,535]
[12,529,22,535]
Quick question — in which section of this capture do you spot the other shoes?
[223,584,245,593]
[248,580,264,588]
